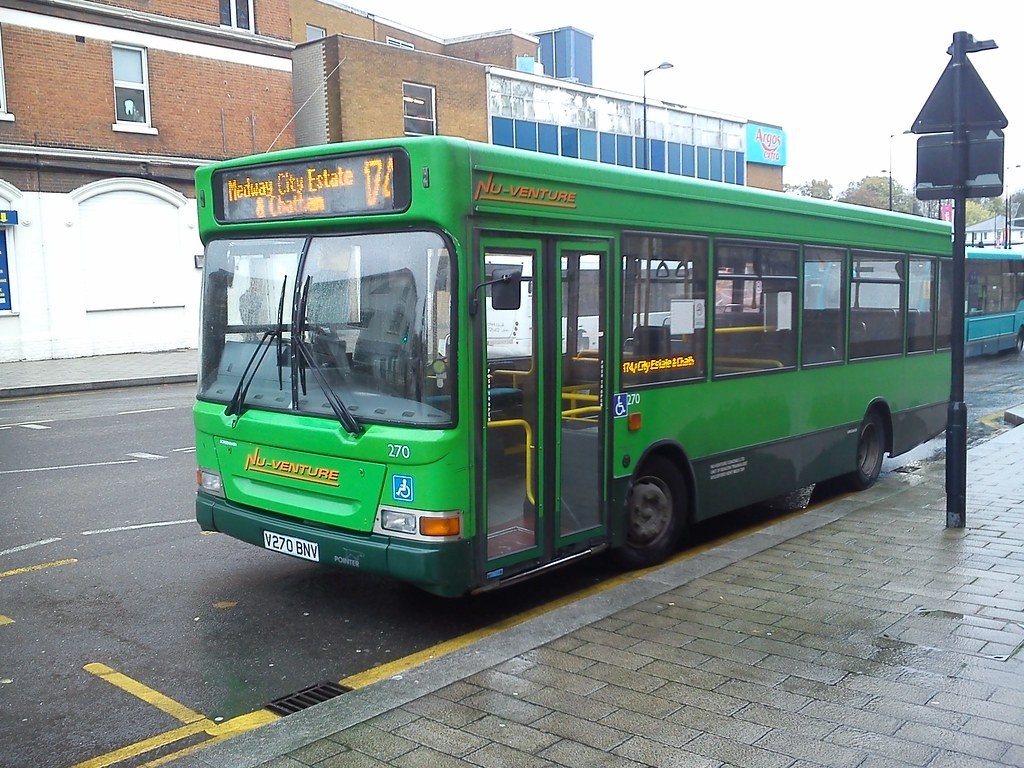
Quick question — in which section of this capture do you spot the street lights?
[1009,165,1021,249]
[880,130,914,212]
[643,62,673,170]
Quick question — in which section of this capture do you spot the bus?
[817,246,1024,360]
[193,137,963,605]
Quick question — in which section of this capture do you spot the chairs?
[404,281,942,433]
